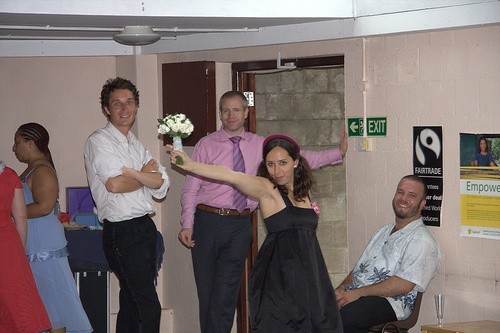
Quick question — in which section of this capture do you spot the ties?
[228,136,247,214]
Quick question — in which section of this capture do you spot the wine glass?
[434,294,445,327]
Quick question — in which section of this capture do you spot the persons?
[334,174,442,333]
[178,92,348,333]
[84,77,171,333]
[12,122,95,333]
[471,136,496,167]
[0,161,53,333]
[166,135,345,332]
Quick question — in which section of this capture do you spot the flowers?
[156,112,194,166]
[311,200,321,214]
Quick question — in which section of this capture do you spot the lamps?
[113,25,161,46]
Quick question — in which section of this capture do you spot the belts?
[196,203,250,216]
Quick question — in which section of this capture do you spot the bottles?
[53,200,60,220]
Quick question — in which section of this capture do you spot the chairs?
[368,291,424,333]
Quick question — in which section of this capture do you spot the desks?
[420,320,500,333]
